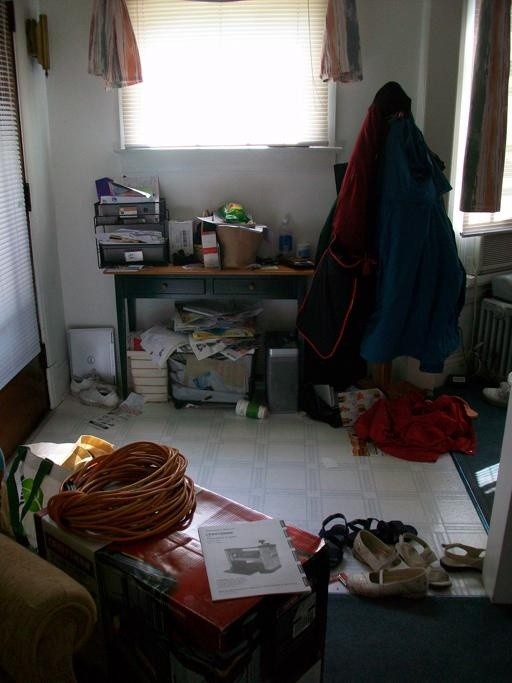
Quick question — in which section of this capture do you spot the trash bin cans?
[264,330,299,415]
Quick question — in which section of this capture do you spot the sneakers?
[70,376,120,408]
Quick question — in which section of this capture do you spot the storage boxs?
[43,482,331,682]
[169,353,255,412]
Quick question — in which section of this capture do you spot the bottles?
[278,217,294,258]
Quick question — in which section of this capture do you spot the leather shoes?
[318,513,487,596]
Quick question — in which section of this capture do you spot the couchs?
[2,536,100,682]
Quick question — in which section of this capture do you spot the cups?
[235,398,269,420]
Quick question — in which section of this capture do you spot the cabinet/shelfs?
[93,198,171,268]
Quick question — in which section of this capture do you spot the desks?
[99,262,317,415]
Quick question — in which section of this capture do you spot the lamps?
[25,14,51,79]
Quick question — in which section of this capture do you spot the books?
[95,174,164,264]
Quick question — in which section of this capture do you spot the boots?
[482,372,512,405]
